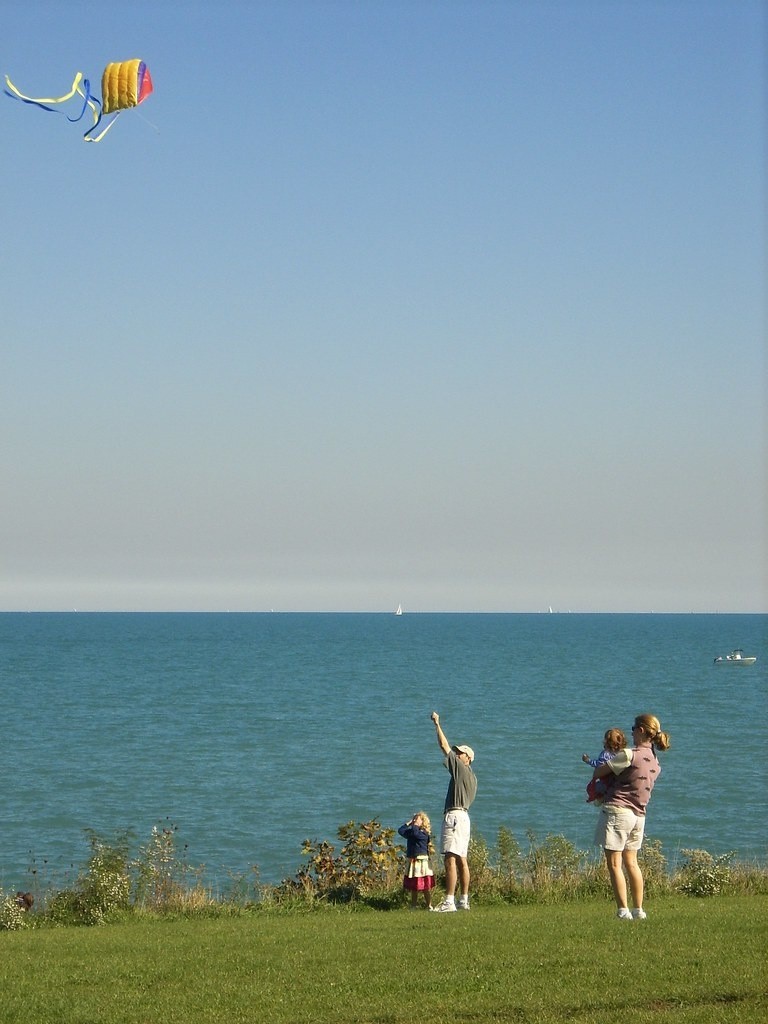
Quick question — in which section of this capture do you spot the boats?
[713,648,757,668]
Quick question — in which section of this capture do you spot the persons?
[583,729,627,802]
[596,714,670,920]
[398,812,435,912]
[430,713,477,912]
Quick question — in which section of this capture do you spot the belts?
[444,808,466,814]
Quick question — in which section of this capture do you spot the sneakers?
[432,901,457,913]
[455,901,470,910]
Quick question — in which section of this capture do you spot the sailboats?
[394,604,403,616]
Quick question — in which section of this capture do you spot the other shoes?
[614,912,633,920]
[426,906,433,912]
[632,911,646,920]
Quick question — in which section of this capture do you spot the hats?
[452,745,475,762]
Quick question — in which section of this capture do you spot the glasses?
[632,725,644,731]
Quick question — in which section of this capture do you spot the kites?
[3,59,153,143]
